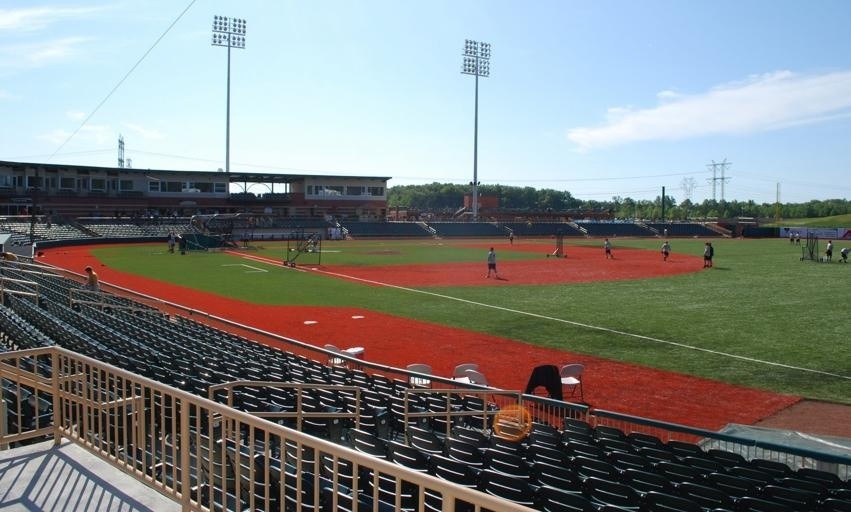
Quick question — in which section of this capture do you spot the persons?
[702,242,713,268]
[485,248,497,278]
[84,266,98,286]
[839,248,851,263]
[244,230,248,247]
[1,252,18,261]
[795,232,801,247]
[790,232,794,245]
[509,231,513,244]
[826,240,832,262]
[167,233,186,255]
[661,241,671,264]
[604,239,613,259]
